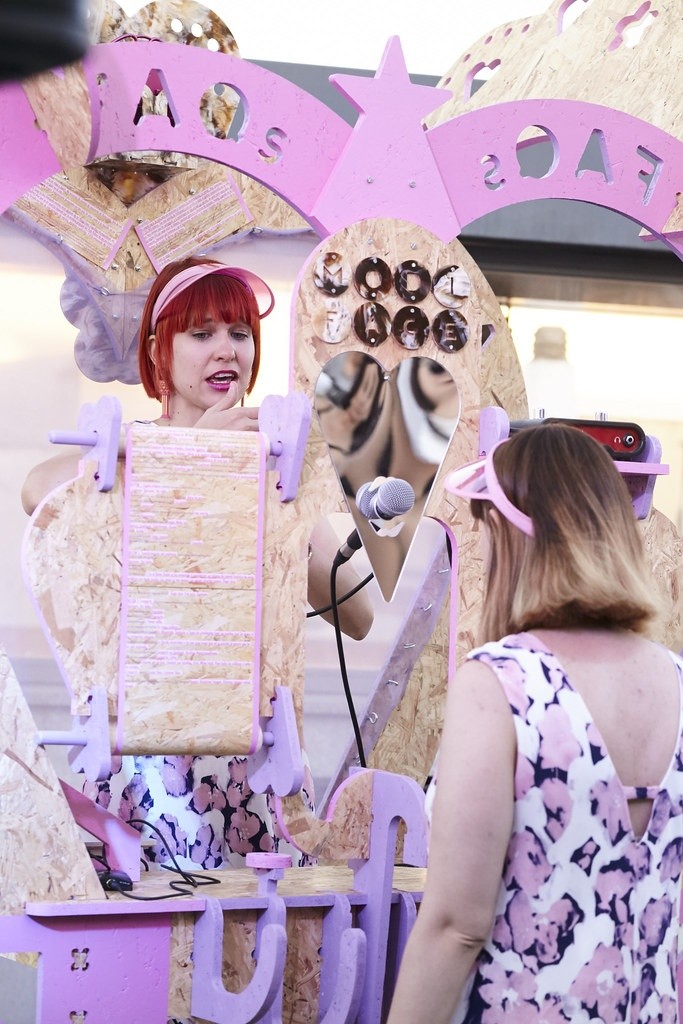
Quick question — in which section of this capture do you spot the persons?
[21,257,374,871]
[386,423,682,1024]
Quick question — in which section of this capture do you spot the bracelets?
[308,542,312,561]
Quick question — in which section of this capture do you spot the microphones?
[332,478,415,568]
[356,481,381,520]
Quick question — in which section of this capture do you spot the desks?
[0,864,426,1024]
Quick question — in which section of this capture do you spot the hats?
[443,437,536,539]
[150,262,275,334]
